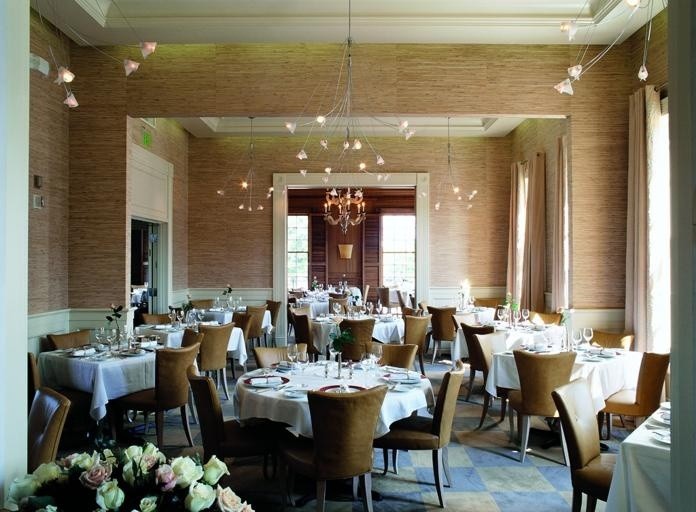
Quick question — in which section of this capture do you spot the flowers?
[3,439,252,512]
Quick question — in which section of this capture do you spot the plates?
[648,398,670,449]
[241,340,421,397]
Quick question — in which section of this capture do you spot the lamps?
[335,242,354,260]
[281,0,417,199]
[213,116,277,215]
[420,115,478,213]
[320,184,368,236]
[34,0,159,110]
[548,0,653,98]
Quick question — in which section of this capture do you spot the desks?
[621,402,671,511]
[296,285,405,361]
[189,307,270,352]
[135,323,243,399]
[491,346,644,451]
[238,360,431,508]
[477,321,566,400]
[431,307,495,355]
[36,343,172,452]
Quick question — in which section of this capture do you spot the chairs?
[185,364,279,493]
[141,299,215,325]
[196,321,236,400]
[551,377,620,511]
[472,331,517,442]
[589,330,635,350]
[460,324,494,407]
[288,285,416,339]
[28,353,98,452]
[277,384,388,511]
[427,305,457,365]
[106,341,200,452]
[47,329,133,423]
[133,328,203,436]
[230,299,281,355]
[512,350,578,467]
[28,385,71,476]
[290,311,331,363]
[520,311,562,325]
[401,306,429,376]
[604,352,670,440]
[335,318,375,362]
[473,297,502,309]
[373,359,465,509]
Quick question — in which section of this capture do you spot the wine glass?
[455,292,480,312]
[56,326,156,359]
[494,304,593,359]
[209,295,246,311]
[289,280,398,323]
[152,309,218,333]
